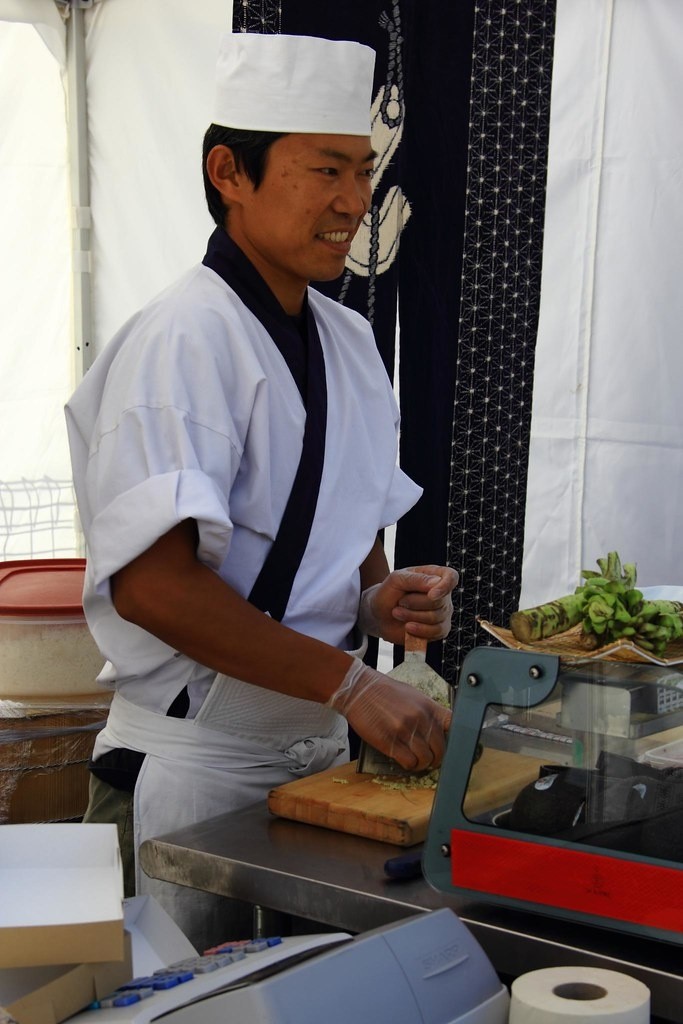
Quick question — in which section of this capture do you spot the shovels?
[359,590,452,778]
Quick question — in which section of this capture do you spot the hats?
[209,34,376,136]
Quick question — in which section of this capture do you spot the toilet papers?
[508,966,652,1024]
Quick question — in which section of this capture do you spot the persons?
[63,33,459,956]
